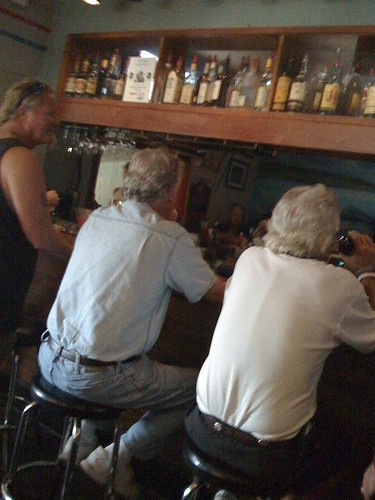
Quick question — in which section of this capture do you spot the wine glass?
[50,124,135,161]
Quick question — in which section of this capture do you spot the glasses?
[16,83,46,108]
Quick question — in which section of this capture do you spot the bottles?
[153,51,375,119]
[63,49,131,102]
[336,235,354,258]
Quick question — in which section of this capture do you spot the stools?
[1,325,40,478]
[0,374,129,500]
[179,433,275,500]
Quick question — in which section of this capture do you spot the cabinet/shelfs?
[48,23,375,154]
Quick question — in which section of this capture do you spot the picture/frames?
[225,158,249,191]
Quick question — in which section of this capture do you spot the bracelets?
[357,272,375,280]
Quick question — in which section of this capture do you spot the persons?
[38,148,247,500]
[186,153,269,239]
[0,79,72,362]
[184,185,375,500]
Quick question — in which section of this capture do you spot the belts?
[47,335,143,366]
[200,411,308,447]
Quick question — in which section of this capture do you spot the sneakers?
[82,444,140,499]
[59,435,95,463]
